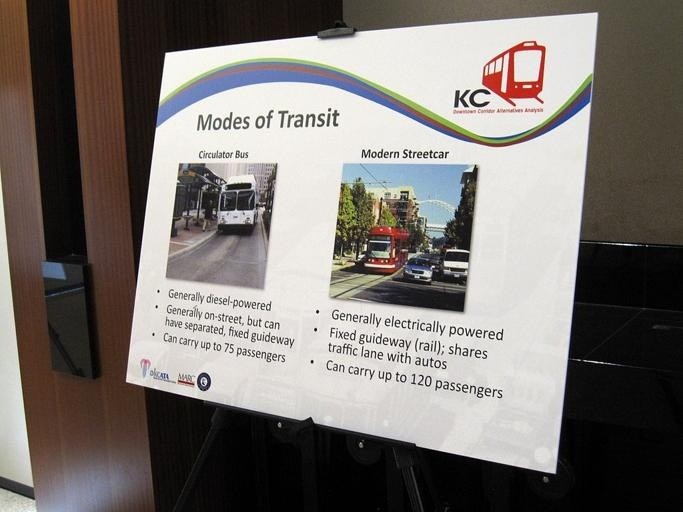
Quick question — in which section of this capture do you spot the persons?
[202,200,213,232]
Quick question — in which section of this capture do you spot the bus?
[216,174,261,234]
[363,225,409,274]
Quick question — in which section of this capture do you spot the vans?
[439,248,470,280]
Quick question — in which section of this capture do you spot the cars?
[402,257,436,284]
